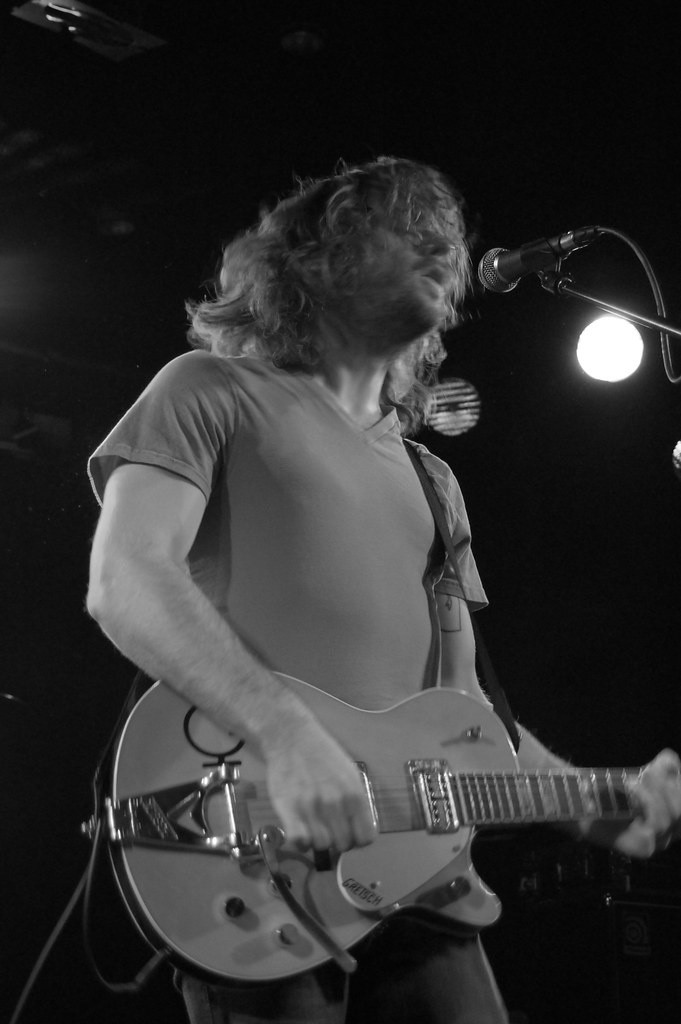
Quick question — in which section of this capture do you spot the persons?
[85,156,680,1024]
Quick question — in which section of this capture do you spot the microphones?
[478,226,605,293]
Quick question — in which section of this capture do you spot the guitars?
[104,666,643,989]
